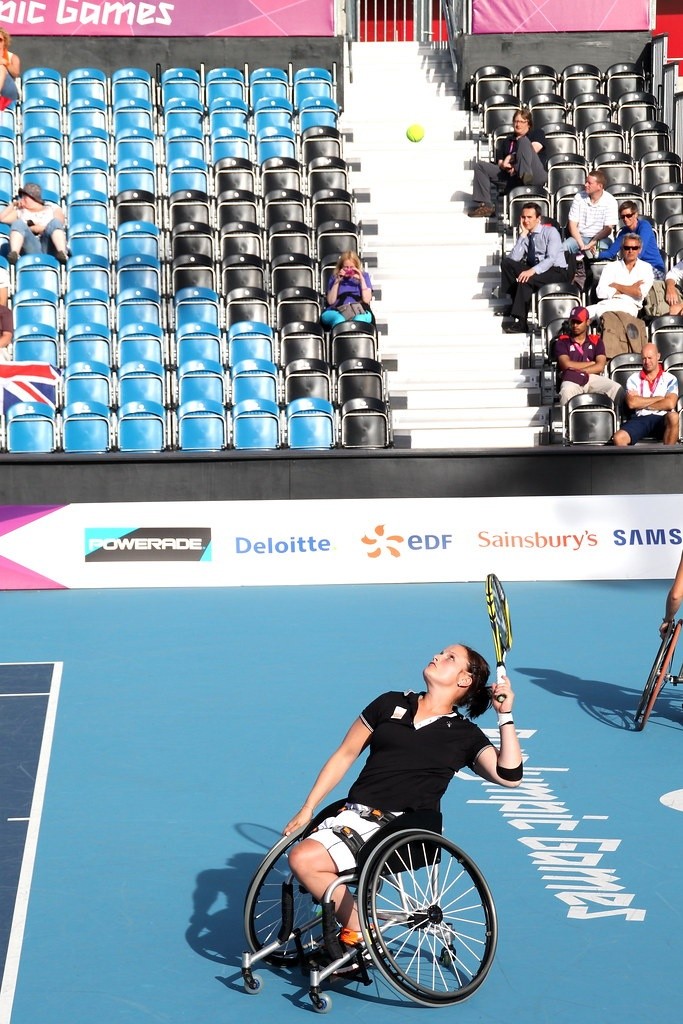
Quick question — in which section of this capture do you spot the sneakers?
[318,923,384,974]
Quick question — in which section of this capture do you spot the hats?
[568,307,589,322]
[18,183,44,204]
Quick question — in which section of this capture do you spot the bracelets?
[497,711,514,728]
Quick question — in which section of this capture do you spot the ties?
[525,233,537,268]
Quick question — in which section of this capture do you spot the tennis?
[407,124,424,143]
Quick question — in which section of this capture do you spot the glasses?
[571,319,582,324]
[623,245,639,250]
[621,212,635,219]
[513,119,527,124]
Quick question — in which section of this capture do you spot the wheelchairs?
[240,798,498,1014]
[636,617,683,730]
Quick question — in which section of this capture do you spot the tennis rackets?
[483,573,514,702]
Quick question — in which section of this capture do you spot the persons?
[554,306,626,408]
[320,251,373,331]
[590,201,665,281]
[468,109,548,217]
[665,259,683,316]
[563,171,619,293]
[585,232,654,325]
[0,304,13,362]
[0,184,68,265]
[495,203,569,333]
[282,644,523,974]
[612,343,679,446]
[659,550,683,640]
[0,27,21,100]
[0,267,9,307]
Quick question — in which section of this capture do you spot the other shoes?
[8,251,20,265]
[468,207,496,218]
[522,172,534,185]
[495,306,511,316]
[56,251,68,264]
[505,318,529,333]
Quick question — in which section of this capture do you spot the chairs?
[0,62,387,456]
[462,63,683,447]
